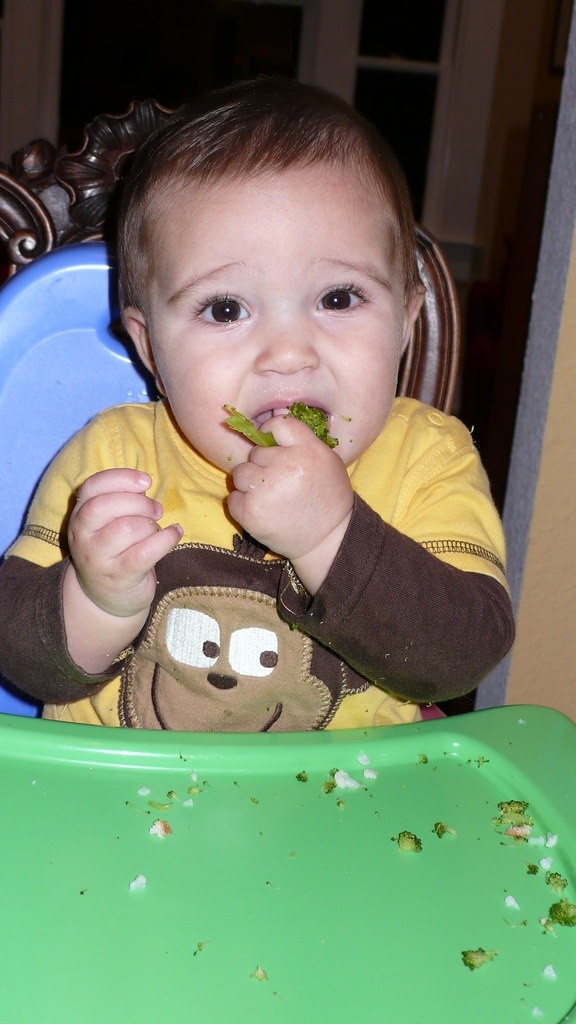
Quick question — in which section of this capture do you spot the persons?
[0,81,515,732]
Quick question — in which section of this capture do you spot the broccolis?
[222,402,340,452]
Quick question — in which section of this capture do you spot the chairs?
[0,98,576,1024]
